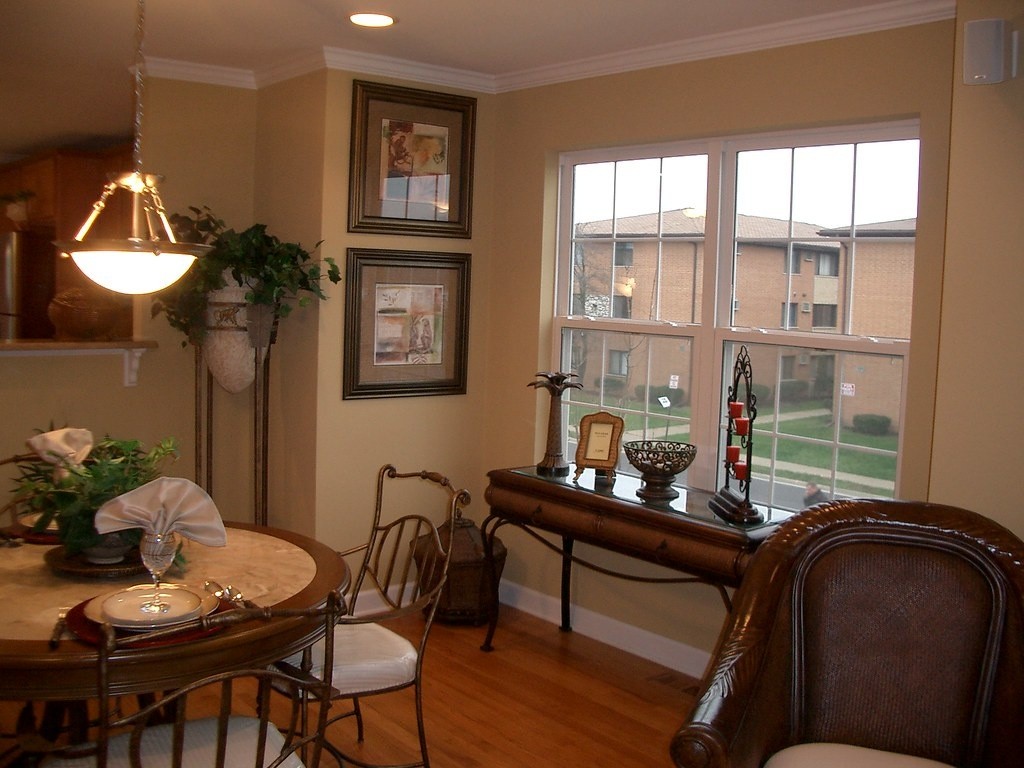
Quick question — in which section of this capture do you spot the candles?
[730,401,744,418]
[735,463,747,478]
[727,446,740,462]
[735,418,749,434]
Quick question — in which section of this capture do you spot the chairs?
[47,591,347,768]
[255,464,471,768]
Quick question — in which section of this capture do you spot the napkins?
[94,475,229,548]
[25,426,94,465]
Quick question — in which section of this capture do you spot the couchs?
[669,498,1024,768]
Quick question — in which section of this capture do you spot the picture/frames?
[341,247,471,401]
[347,79,478,239]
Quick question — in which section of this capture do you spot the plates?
[20,512,64,532]
[84,584,219,628]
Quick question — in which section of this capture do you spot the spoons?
[226,583,260,609]
[205,581,245,614]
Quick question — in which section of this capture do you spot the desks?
[0,521,353,746]
[479,462,778,654]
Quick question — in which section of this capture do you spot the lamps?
[52,0,215,296]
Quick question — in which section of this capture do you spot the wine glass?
[139,534,177,613]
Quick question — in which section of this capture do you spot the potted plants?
[145,205,340,395]
[8,432,192,573]
